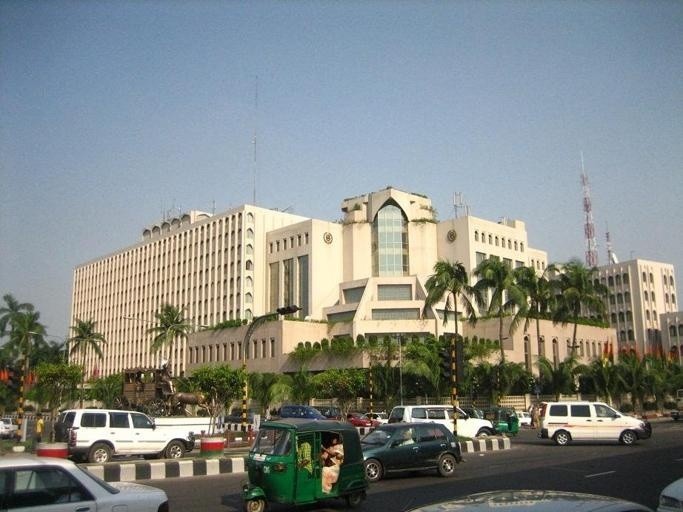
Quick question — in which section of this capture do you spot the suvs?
[55,408,195,463]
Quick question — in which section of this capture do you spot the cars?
[388,404,495,437]
[517,411,532,426]
[360,422,461,483]
[0,456,170,512]
[281,406,327,420]
[0,418,19,439]
[404,477,683,512]
[330,413,389,426]
[225,411,256,424]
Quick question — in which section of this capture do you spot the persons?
[288,437,313,480]
[33,411,45,441]
[320,436,344,495]
[526,401,543,430]
[393,428,414,449]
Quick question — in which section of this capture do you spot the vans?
[538,401,651,445]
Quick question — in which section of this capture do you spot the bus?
[676,389,683,411]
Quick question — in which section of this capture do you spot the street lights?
[392,332,406,405]
[26,330,100,406]
[123,315,209,362]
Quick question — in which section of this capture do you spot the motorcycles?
[240,418,370,512]
[483,407,519,436]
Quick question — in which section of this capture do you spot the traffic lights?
[276,305,297,315]
